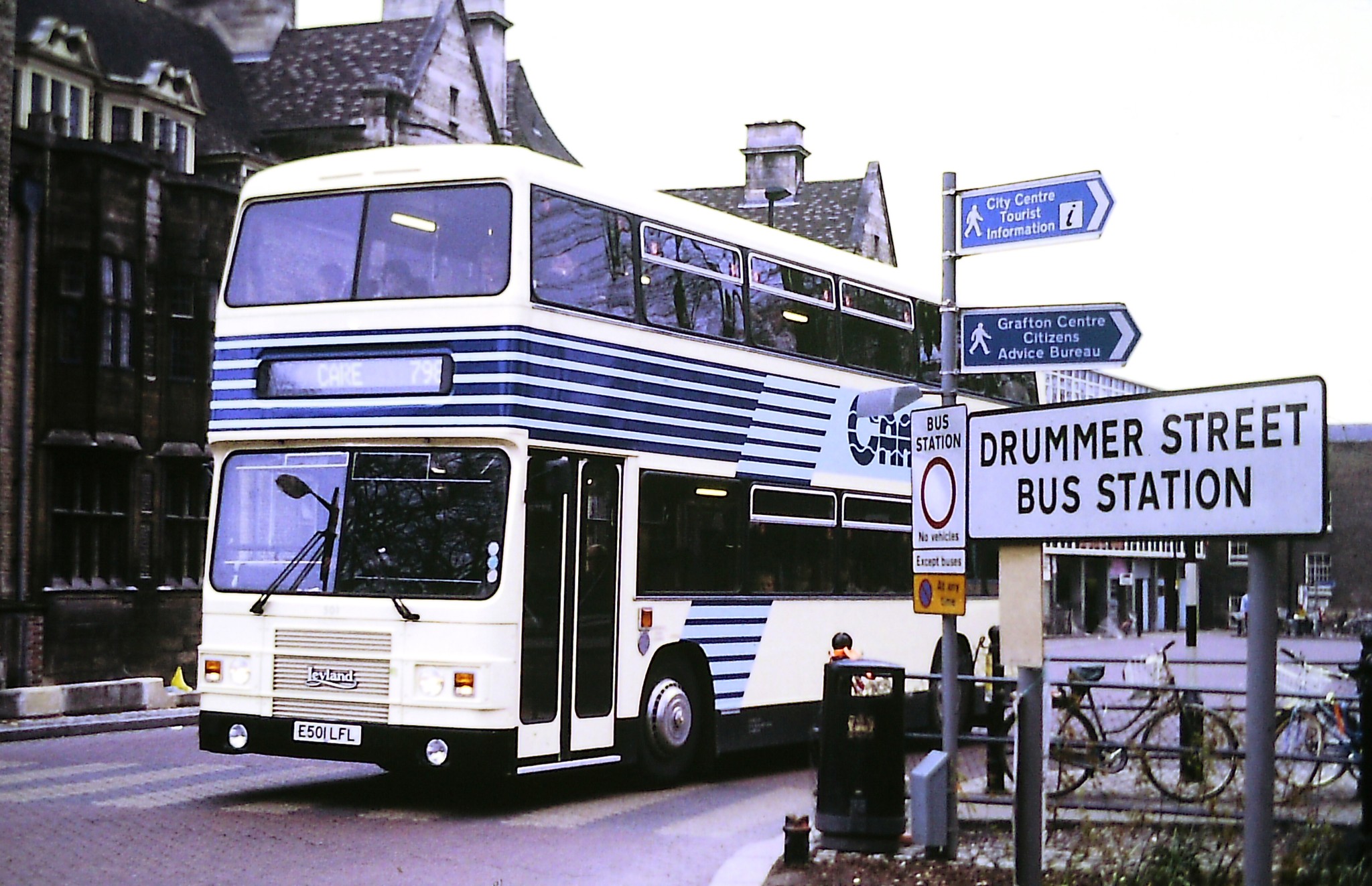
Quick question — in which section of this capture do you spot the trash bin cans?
[817,658,911,856]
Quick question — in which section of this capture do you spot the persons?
[1237,589,1248,637]
[1353,607,1363,634]
[1353,631,1372,836]
[317,262,347,301]
[1338,607,1347,638]
[1313,606,1330,637]
[1290,603,1308,637]
[373,259,413,299]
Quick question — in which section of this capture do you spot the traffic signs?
[969,382,1340,547]
[959,168,1110,256]
[959,302,1141,370]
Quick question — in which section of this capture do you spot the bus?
[193,146,1013,787]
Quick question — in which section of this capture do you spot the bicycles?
[1276,648,1366,796]
[996,640,1242,803]
